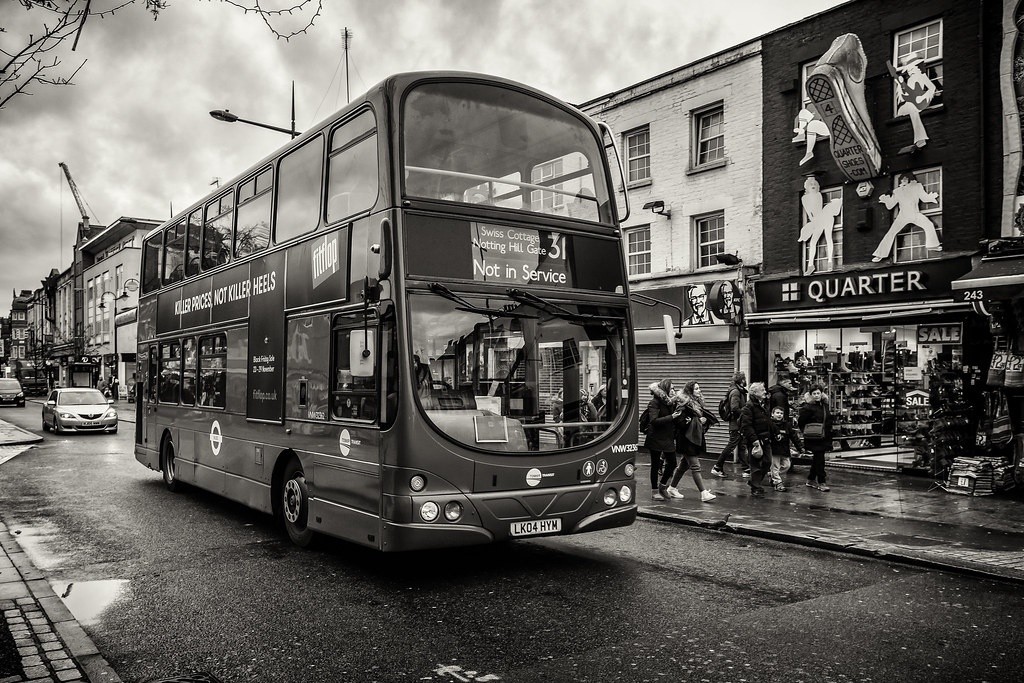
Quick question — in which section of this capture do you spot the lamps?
[643,201,671,220]
[801,170,824,177]
[715,254,742,265]
[896,145,921,156]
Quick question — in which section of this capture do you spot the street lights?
[98,291,118,378]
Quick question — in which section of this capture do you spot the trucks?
[14,361,49,395]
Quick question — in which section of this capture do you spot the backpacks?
[719,388,741,422]
[638,398,659,434]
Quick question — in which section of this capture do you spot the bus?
[133,70,680,555]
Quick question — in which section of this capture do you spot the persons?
[96,373,119,400]
[712,373,836,494]
[549,382,607,448]
[643,380,717,503]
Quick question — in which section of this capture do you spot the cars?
[0,378,25,407]
[42,388,119,433]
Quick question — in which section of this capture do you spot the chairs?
[169,247,230,283]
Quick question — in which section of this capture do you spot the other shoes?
[775,350,895,449]
[767,472,775,487]
[805,33,881,181]
[742,469,751,478]
[710,465,727,478]
[806,479,819,488]
[666,485,684,498]
[774,483,787,492]
[747,480,765,493]
[818,483,830,491]
[701,489,716,502]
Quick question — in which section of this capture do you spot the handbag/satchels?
[804,423,826,439]
[986,352,1007,386]
[974,392,1013,448]
[1004,354,1024,387]
[752,445,763,459]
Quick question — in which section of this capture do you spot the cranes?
[58,161,97,244]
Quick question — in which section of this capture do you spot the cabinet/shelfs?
[927,371,964,476]
[829,369,928,440]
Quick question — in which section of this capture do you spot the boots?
[659,482,671,499]
[651,489,664,500]
